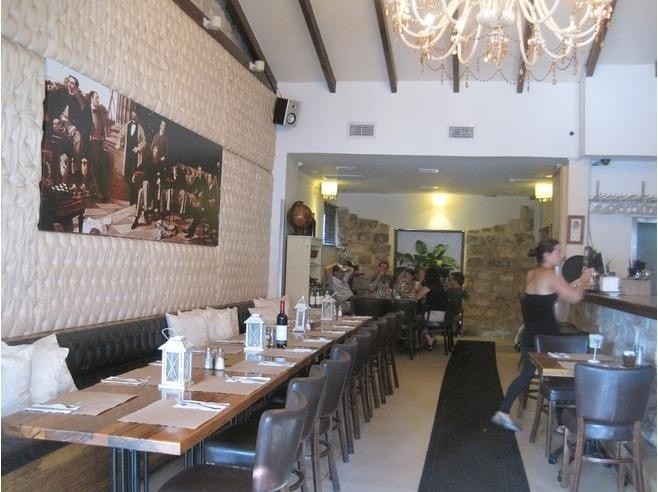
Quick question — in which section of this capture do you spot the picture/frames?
[567,215,585,245]
[394,228,465,287]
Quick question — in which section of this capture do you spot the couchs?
[1,300,255,492]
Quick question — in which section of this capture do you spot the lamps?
[384,0,614,92]
[249,60,265,72]
[202,15,222,31]
[534,182,553,203]
[321,182,338,201]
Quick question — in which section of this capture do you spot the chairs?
[517,334,656,492]
[157,296,405,491]
[351,296,463,361]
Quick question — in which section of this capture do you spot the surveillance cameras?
[600,158,611,164]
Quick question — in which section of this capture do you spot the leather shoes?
[490,414,521,431]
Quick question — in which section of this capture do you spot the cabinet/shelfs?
[285,235,322,320]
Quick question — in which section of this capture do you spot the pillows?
[1,333,82,463]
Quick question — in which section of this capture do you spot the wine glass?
[591,194,656,217]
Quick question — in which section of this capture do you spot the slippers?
[428,338,437,351]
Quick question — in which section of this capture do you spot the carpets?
[417,340,531,492]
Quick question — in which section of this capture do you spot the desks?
[1,365,290,492]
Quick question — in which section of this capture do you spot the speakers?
[273,97,300,127]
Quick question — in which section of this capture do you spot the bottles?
[634,345,642,366]
[268,328,275,348]
[203,346,225,370]
[338,305,342,316]
[310,282,330,307]
[276,300,288,349]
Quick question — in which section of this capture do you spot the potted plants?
[394,240,459,290]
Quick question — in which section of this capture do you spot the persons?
[44,76,223,238]
[489,238,593,433]
[321,262,357,302]
[414,267,465,352]
[367,261,393,292]
[395,267,420,294]
